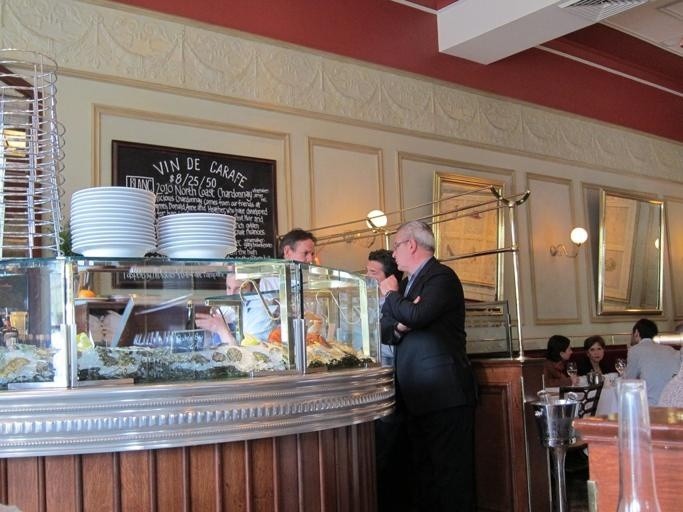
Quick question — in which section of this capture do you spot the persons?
[258,228,327,343]
[623,319,683,408]
[195,273,276,374]
[543,334,571,399]
[339,249,404,369]
[577,334,616,384]
[377,219,482,512]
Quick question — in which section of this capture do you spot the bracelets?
[384,290,394,298]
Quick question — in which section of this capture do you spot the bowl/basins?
[109,295,132,302]
[73,297,110,306]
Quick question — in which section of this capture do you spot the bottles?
[613,378,662,511]
[538,390,553,405]
[563,392,578,404]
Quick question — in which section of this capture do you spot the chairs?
[554,382,603,450]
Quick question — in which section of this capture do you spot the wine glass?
[565,360,579,386]
[614,359,626,376]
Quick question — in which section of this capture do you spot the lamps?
[551,228,587,261]
[346,210,387,248]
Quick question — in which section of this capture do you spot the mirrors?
[431,170,504,314]
[596,188,665,318]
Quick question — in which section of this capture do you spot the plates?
[67,185,158,260]
[155,211,237,260]
